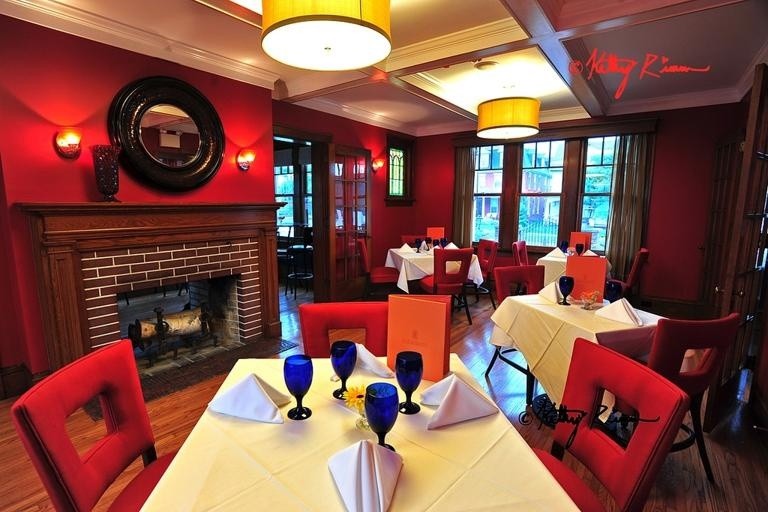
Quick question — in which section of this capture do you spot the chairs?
[529,335,692,511]
[297,299,388,356]
[10,335,184,512]
[284,226,314,300]
[613,310,741,484]
[355,234,648,325]
[484,266,545,404]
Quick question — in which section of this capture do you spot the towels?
[326,437,404,511]
[207,370,292,425]
[417,372,499,430]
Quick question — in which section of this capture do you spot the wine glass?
[557,275,575,306]
[575,243,584,256]
[559,240,569,253]
[415,237,448,253]
[605,280,623,304]
[363,382,397,452]
[328,340,357,401]
[283,353,313,420]
[355,404,372,432]
[394,350,424,415]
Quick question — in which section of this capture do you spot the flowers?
[340,382,366,429]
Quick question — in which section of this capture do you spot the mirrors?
[107,73,225,196]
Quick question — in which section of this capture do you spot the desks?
[138,352,582,511]
[488,290,673,428]
[276,223,310,294]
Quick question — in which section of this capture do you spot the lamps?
[260,1,392,73]
[54,126,83,159]
[476,96,542,141]
[236,148,257,172]
[371,157,385,172]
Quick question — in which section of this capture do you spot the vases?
[92,142,122,203]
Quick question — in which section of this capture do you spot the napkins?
[594,297,644,326]
[539,281,570,303]
